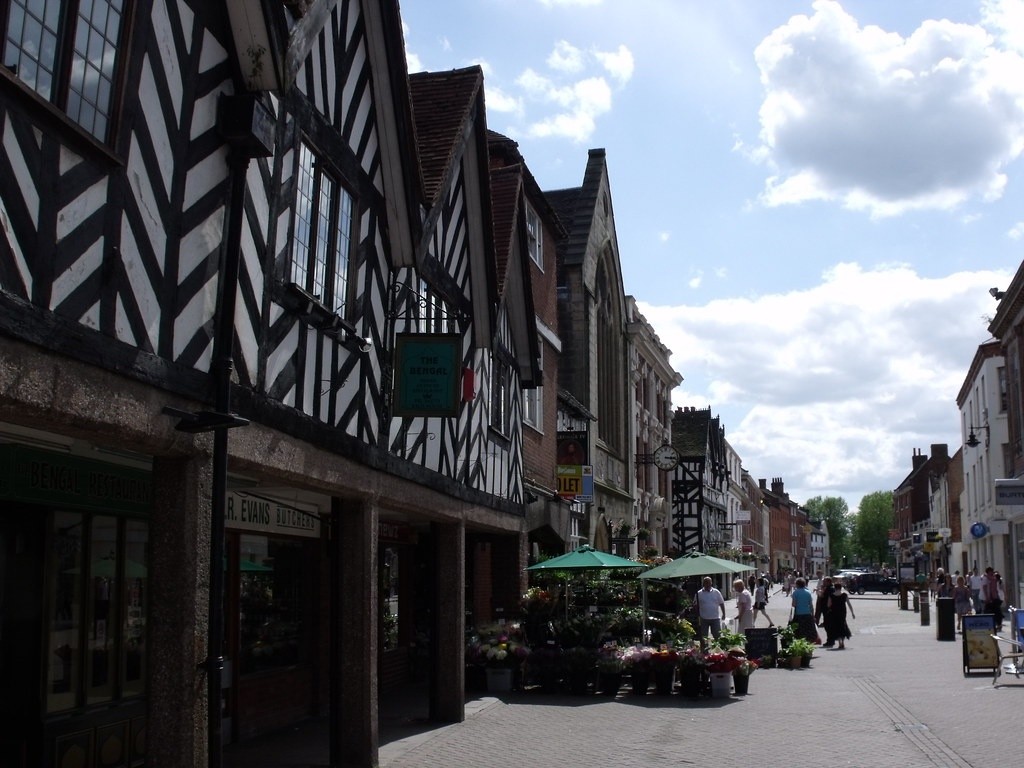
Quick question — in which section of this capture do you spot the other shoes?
[815,639,821,644]
[769,624,774,627]
[838,645,844,650]
[957,624,960,629]
[823,642,834,646]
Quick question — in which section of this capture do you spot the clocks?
[653,444,678,471]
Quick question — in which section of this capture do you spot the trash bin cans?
[936,597,956,642]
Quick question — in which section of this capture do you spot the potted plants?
[777,623,799,649]
[788,642,802,669]
[801,640,816,667]
[535,551,697,643]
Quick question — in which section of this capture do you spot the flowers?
[648,651,678,671]
[593,643,628,671]
[703,649,737,672]
[734,658,759,675]
[469,624,528,669]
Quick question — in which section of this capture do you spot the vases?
[653,668,677,695]
[733,673,748,694]
[710,673,731,699]
[598,672,622,697]
[469,664,512,692]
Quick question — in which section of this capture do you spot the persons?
[693,576,725,656]
[752,578,774,628]
[791,578,821,645]
[813,570,855,650]
[928,572,937,599]
[970,567,982,614]
[937,568,944,597]
[938,574,955,597]
[954,576,972,633]
[733,579,755,634]
[979,567,1007,636]
[955,571,960,586]
[916,570,926,584]
[748,571,772,596]
[781,568,810,597]
[966,570,973,597]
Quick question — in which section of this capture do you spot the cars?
[844,573,899,595]
[834,571,859,586]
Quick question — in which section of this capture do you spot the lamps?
[965,424,990,447]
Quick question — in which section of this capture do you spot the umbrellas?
[523,544,649,621]
[636,546,759,645]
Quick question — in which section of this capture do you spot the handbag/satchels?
[720,619,727,630]
